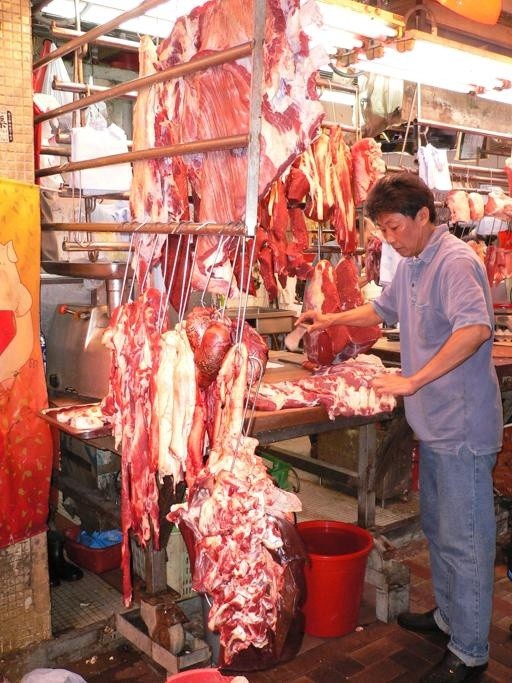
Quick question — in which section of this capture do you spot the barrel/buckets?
[293,521,373,639]
[166,669,236,683]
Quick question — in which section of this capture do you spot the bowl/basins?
[379,137,416,155]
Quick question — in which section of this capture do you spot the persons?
[292,165,505,682]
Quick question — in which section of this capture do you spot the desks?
[36,350,427,679]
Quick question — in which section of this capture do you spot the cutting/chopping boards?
[371,335,512,370]
[243,349,403,436]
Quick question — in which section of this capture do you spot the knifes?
[283,321,311,351]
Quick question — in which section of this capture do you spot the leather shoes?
[419,649,488,683]
[397,610,447,634]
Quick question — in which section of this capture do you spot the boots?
[51,573,61,586]
[50,530,84,582]
